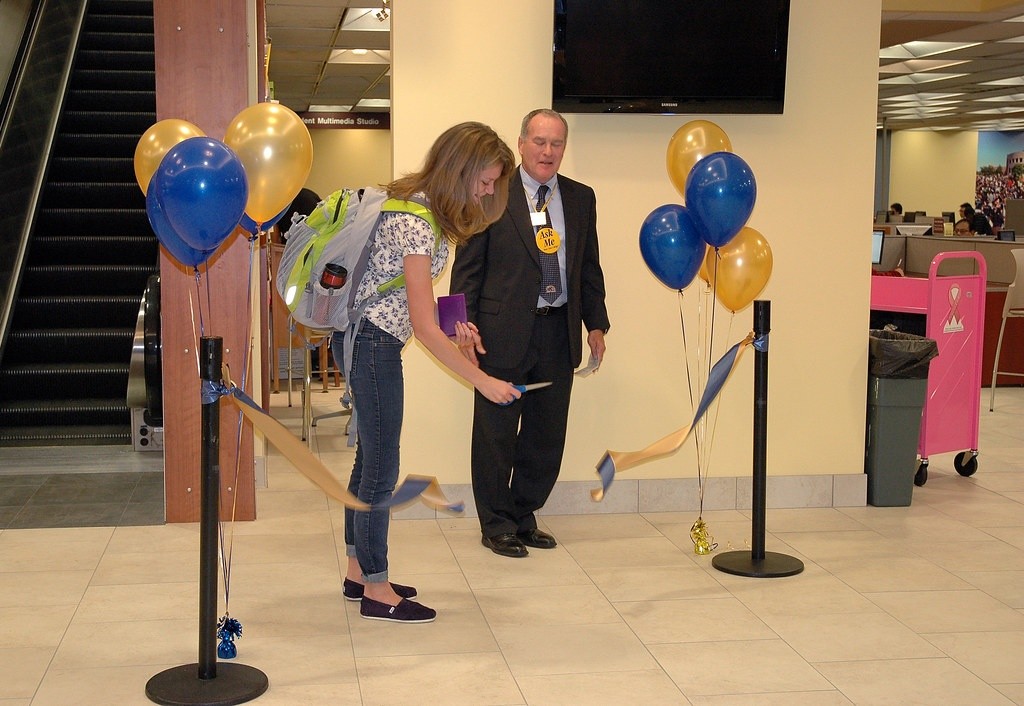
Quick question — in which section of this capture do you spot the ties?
[535,186,563,304]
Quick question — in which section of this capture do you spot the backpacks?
[276,186,440,351]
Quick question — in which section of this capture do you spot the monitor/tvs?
[875,210,889,223]
[552,0,790,114]
[904,212,915,222]
[942,211,955,224]
[871,228,885,265]
[895,225,932,236]
[997,230,1015,241]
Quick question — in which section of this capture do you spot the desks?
[270,242,328,394]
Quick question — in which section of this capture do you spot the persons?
[330,121,524,623]
[953,203,1004,236]
[890,203,902,215]
[450,109,611,556]
[975,172,1024,214]
[870,266,904,278]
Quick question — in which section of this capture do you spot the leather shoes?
[516,529,557,548]
[481,534,529,557]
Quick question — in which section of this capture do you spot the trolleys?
[871,250,987,486]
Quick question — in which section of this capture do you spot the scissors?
[498,382,553,405]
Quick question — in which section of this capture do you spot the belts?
[535,306,567,316]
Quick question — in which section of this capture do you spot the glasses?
[954,228,970,234]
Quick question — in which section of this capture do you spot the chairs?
[989,248,1024,413]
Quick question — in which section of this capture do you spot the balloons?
[665,119,732,198]
[685,152,757,248]
[134,102,313,277]
[699,226,773,313]
[639,203,707,291]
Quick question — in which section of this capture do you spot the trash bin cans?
[864,328,937,507]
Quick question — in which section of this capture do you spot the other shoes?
[359,595,437,623]
[343,576,417,600]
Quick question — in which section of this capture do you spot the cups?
[313,263,347,325]
